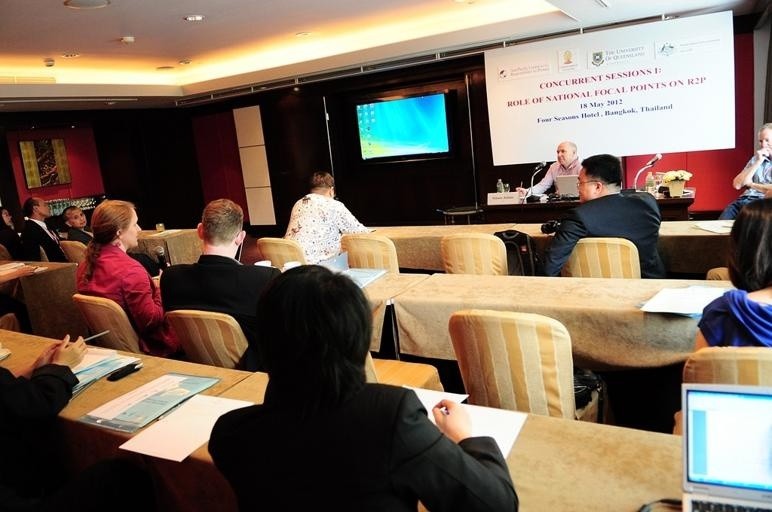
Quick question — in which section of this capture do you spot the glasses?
[576,179,601,189]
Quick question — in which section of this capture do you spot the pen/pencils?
[157,406,182,422]
[440,411,447,414]
[83,331,111,342]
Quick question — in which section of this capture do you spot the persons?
[283,171,374,264]
[544,154,666,278]
[208,266,519,512]
[160,199,280,342]
[515,142,584,200]
[719,123,772,219]
[0,207,21,259]
[22,197,66,262]
[673,198,772,435]
[62,206,93,245]
[0,334,88,512]
[75,199,178,351]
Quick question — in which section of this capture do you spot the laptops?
[679,378,771,512]
[555,174,579,198]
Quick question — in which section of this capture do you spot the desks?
[122,327,684,512]
[364,219,737,277]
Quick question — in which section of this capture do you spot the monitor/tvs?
[356,89,459,167]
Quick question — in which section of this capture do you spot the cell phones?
[108,362,144,381]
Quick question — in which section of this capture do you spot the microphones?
[534,161,548,170]
[153,244,170,268]
[647,153,665,167]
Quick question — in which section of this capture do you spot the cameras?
[540,219,561,233]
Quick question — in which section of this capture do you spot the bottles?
[44,198,70,217]
[496,179,503,193]
[645,172,654,194]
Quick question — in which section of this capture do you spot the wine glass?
[652,175,663,197]
[503,184,510,192]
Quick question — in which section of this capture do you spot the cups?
[156,223,165,232]
